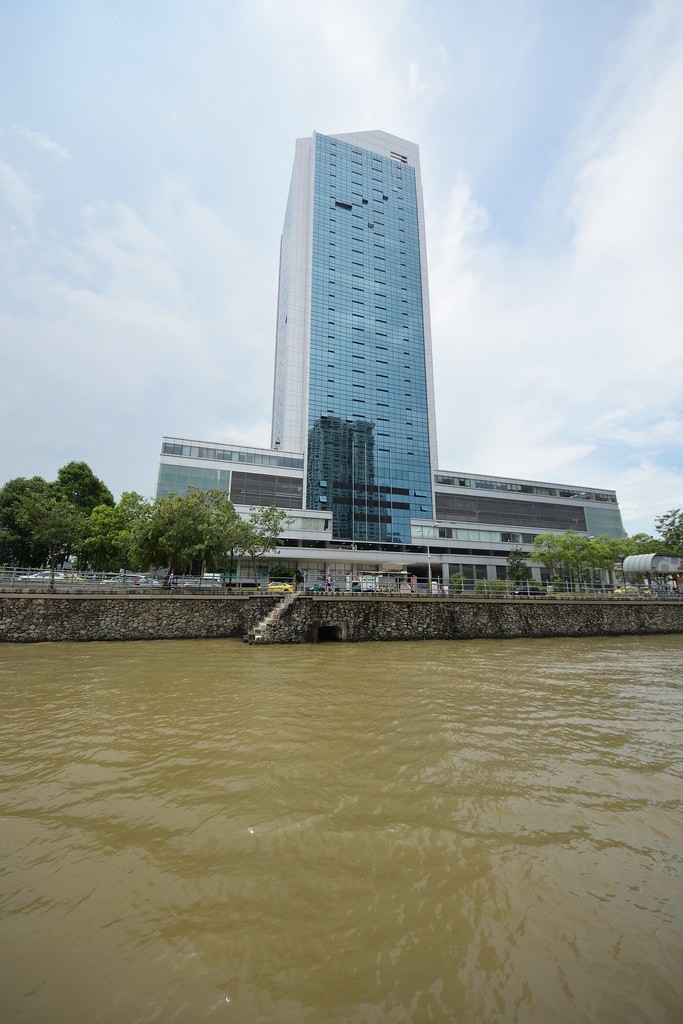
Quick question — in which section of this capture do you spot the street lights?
[427,523,438,592]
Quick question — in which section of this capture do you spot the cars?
[224,581,261,590]
[510,586,546,595]
[256,582,294,592]
[305,583,340,591]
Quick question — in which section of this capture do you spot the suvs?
[100,573,148,584]
[15,571,68,581]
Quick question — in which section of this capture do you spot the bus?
[345,570,408,592]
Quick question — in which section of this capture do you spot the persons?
[326,574,334,593]
